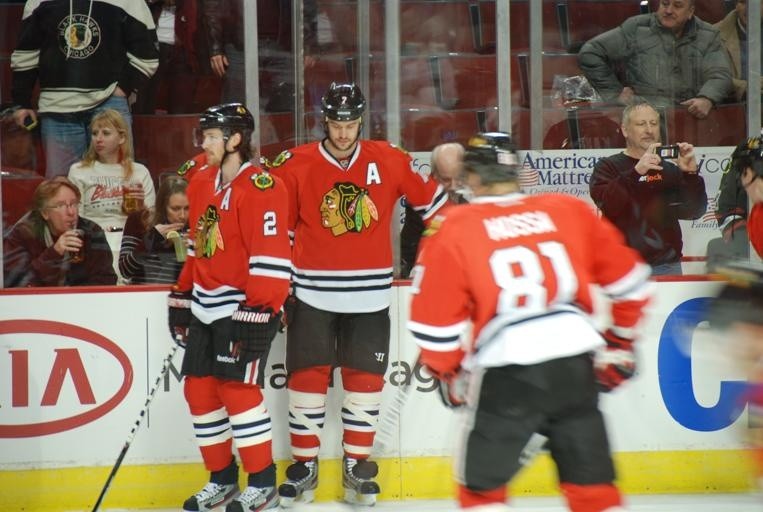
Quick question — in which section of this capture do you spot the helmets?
[196,101,257,131]
[459,130,521,180]
[318,81,367,123]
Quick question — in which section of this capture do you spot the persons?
[588,97,707,275]
[739,168,762,259]
[400,142,464,279]
[10,1,159,175]
[118,173,192,286]
[715,0,762,103]
[269,83,452,498]
[67,106,156,286]
[177,153,209,181]
[166,102,291,511]
[576,0,733,120]
[195,1,325,112]
[706,270,763,501]
[3,177,116,288]
[541,73,626,150]
[706,132,761,271]
[314,0,530,146]
[410,133,659,510]
[132,0,202,114]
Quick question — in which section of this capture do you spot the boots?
[338,454,383,494]
[222,480,282,512]
[183,473,242,512]
[276,456,321,497]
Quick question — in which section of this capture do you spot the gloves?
[167,291,195,349]
[431,364,469,412]
[591,329,637,394]
[225,305,280,365]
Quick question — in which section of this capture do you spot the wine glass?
[67,230,86,263]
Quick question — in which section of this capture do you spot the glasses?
[43,202,82,209]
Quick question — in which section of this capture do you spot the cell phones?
[24,114,37,130]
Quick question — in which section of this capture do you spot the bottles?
[124,172,143,215]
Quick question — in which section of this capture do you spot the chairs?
[134,69,294,188]
[1,112,48,232]
[301,1,747,151]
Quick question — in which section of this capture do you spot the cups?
[169,231,189,262]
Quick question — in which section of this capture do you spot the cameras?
[656,144,678,159]
